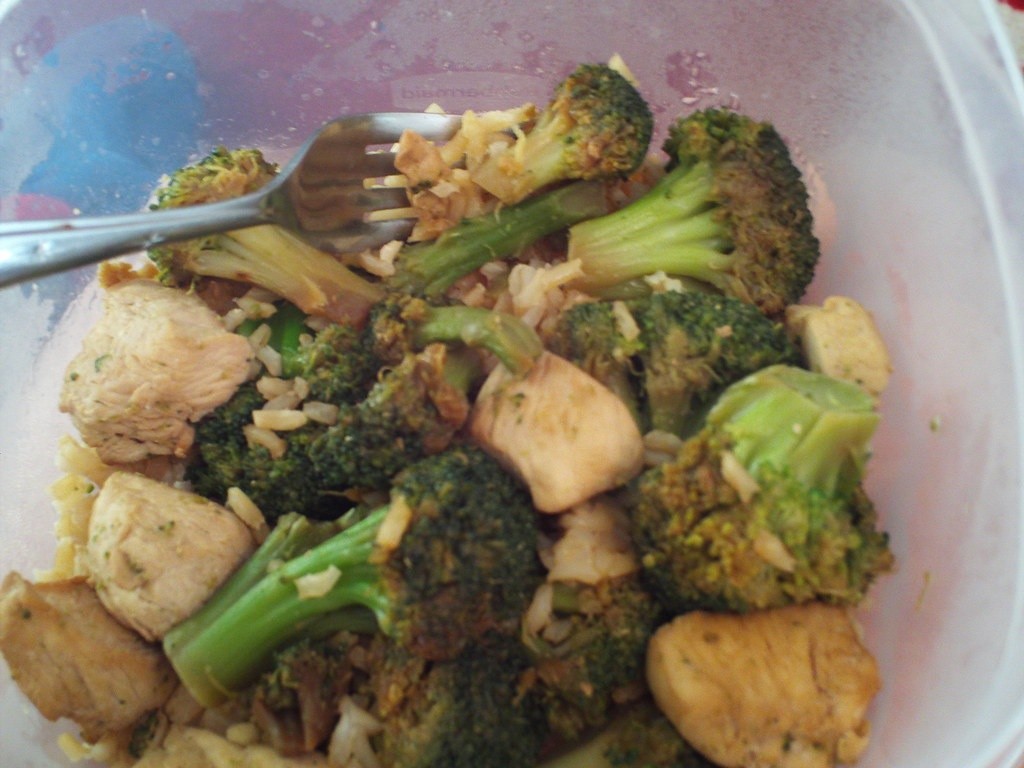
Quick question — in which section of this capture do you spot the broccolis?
[151,65,897,768]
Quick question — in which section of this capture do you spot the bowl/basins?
[1,0,1024,768]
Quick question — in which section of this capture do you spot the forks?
[0,108,523,287]
